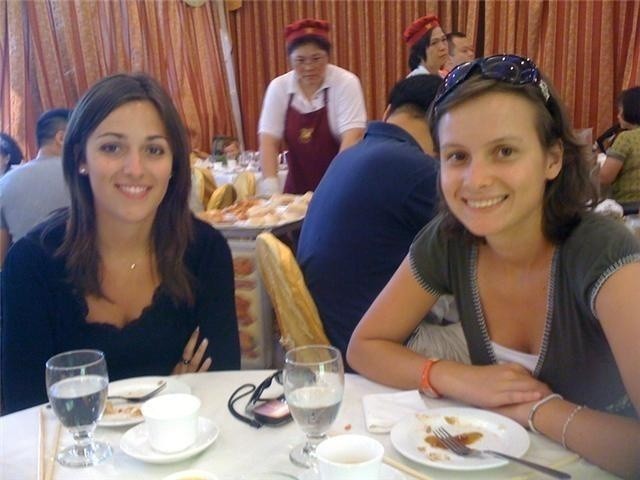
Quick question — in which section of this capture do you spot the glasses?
[245,365,316,414]
[432,54,563,136]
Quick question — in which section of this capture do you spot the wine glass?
[45,349,117,469]
[283,344,346,468]
[238,151,260,173]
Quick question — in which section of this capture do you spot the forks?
[430,424,574,476]
[98,379,168,403]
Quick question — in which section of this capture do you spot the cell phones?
[253,395,291,425]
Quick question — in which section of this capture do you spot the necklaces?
[95,239,152,269]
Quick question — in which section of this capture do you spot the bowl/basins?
[315,434,385,474]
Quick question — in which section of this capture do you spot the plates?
[390,406,531,471]
[118,408,220,464]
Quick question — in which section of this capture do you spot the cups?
[140,395,201,453]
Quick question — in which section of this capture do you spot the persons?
[1,106,71,265]
[258,18,368,202]
[597,85,640,218]
[0,71,241,418]
[346,53,639,480]
[294,73,472,375]
[402,14,474,79]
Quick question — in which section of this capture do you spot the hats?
[284,18,331,45]
[403,15,439,49]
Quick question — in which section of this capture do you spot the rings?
[179,355,190,362]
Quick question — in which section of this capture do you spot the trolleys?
[200,199,307,368]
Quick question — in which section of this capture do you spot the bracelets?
[562,404,586,449]
[418,358,443,399]
[527,393,564,434]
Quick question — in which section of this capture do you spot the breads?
[188,151,256,210]
[240,192,313,225]
[196,202,256,223]
